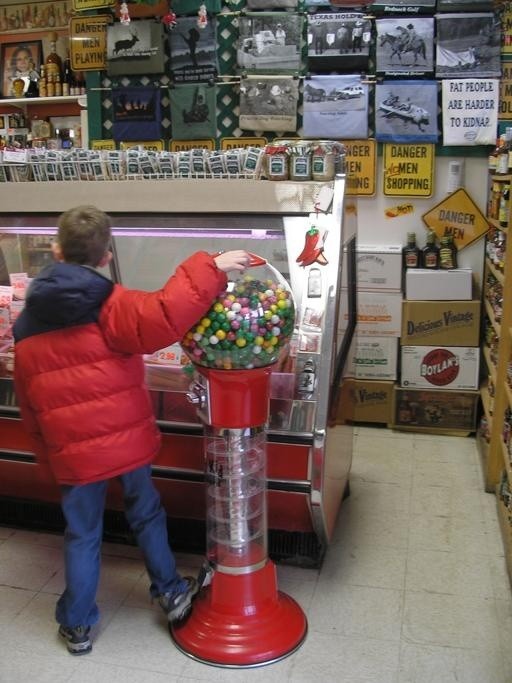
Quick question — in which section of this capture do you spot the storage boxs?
[350,243,402,291]
[395,383,478,431]
[342,337,401,379]
[404,345,480,390]
[334,381,395,426]
[401,267,475,302]
[350,291,405,335]
[400,301,481,347]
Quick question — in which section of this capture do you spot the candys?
[182,275,295,370]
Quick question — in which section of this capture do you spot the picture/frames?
[0,39,45,100]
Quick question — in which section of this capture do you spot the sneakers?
[160,576,200,624]
[59,623,92,654]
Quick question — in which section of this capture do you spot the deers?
[112,27,140,56]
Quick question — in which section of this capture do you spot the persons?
[12,204,250,656]
[383,90,412,112]
[275,23,286,46]
[7,46,37,96]
[336,22,350,54]
[311,20,325,55]
[394,23,416,54]
[351,20,363,52]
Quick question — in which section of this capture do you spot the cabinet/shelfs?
[476,166,511,583]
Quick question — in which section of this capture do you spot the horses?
[305,84,326,103]
[380,32,431,68]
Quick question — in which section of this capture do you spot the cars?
[327,91,350,100]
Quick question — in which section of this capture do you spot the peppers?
[296,225,328,266]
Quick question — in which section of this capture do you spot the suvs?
[341,85,364,94]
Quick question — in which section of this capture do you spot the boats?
[237,36,300,70]
[379,97,430,124]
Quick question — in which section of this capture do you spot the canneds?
[32,137,62,149]
[261,140,335,181]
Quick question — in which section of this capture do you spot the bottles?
[398,396,412,424]
[401,225,458,269]
[1,1,72,31]
[38,40,86,96]
[485,131,512,277]
